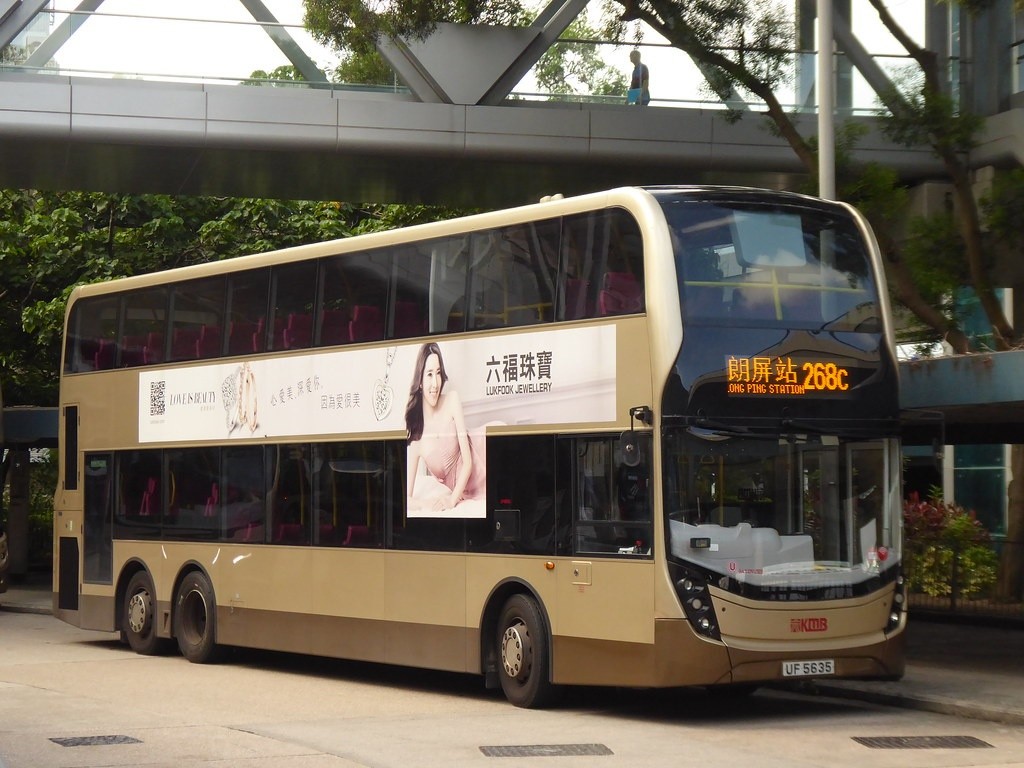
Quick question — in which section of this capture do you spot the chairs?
[93,271,639,373]
[120,470,405,547]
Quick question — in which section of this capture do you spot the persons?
[406,343,486,511]
[625,51,650,106]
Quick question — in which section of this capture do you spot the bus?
[52,185,945,708]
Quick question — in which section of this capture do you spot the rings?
[223,362,256,432]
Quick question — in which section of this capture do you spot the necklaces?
[372,347,397,420]
